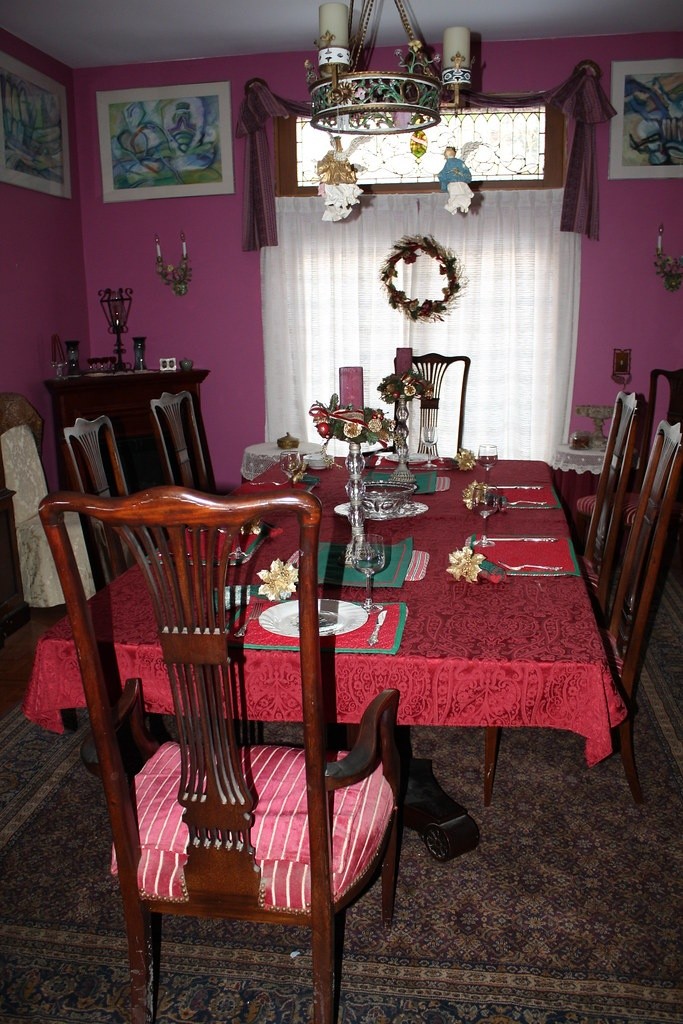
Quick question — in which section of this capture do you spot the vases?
[337,443,380,564]
[389,399,417,486]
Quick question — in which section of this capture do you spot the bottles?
[132,337,147,370]
[64,340,80,375]
[570,430,593,450]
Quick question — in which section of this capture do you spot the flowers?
[376,368,435,407]
[461,479,490,511]
[308,395,406,451]
[382,234,467,324]
[447,544,486,583]
[256,555,300,601]
[453,448,476,472]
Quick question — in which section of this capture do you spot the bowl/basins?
[345,482,417,517]
[303,454,334,469]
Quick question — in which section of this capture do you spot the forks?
[507,500,547,506]
[234,602,263,637]
[497,560,564,571]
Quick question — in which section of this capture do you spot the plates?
[259,599,369,637]
[385,453,438,465]
[333,502,429,521]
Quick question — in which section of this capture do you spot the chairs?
[37,484,400,1024]
[150,390,215,495]
[575,390,648,625]
[61,416,153,587]
[598,418,683,804]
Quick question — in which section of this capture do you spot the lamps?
[306,0,471,136]
[652,225,683,292]
[155,233,192,298]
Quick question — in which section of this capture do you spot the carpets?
[0,516,683,1024]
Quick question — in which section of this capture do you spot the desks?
[548,446,606,522]
[43,368,218,496]
[24,444,627,807]
[239,439,321,484]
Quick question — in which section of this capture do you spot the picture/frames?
[94,81,236,205]
[0,51,73,199]
[609,57,683,182]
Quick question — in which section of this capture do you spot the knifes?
[486,536,559,543]
[486,485,543,490]
[374,456,383,466]
[368,610,387,645]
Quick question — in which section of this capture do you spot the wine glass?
[420,426,438,468]
[280,450,301,489]
[473,484,500,547]
[478,444,498,486]
[351,534,385,614]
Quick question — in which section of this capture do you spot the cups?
[179,360,192,371]
